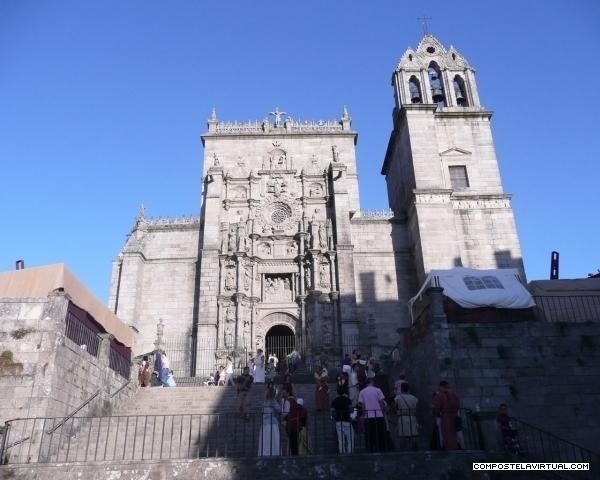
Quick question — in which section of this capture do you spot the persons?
[136,350,176,388]
[210,345,466,456]
[495,403,521,460]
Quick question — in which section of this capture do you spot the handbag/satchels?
[455,416,463,432]
